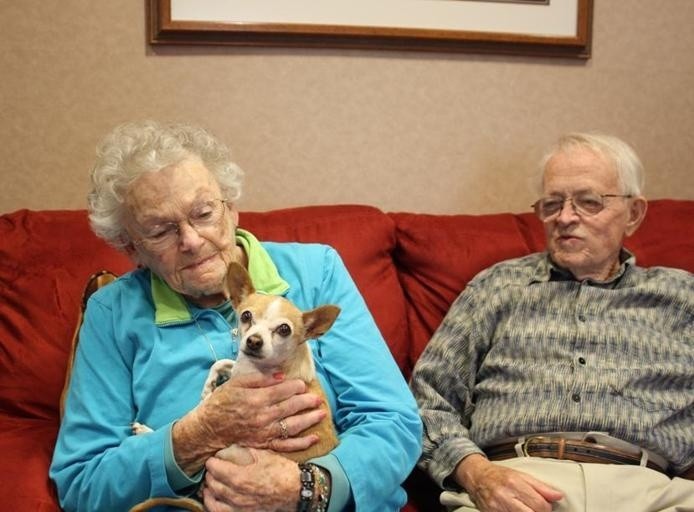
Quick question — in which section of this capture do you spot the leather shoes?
[483,437,672,475]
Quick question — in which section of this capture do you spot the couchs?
[0,198,694,512]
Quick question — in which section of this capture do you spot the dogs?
[128,261,341,512]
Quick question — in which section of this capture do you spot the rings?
[276,420,291,441]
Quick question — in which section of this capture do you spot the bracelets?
[296,462,315,512]
[311,464,328,512]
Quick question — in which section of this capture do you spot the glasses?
[531,188,632,223]
[125,198,232,253]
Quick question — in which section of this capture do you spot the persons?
[410,130,694,512]
[47,111,425,512]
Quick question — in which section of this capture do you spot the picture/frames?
[144,1,596,63]
